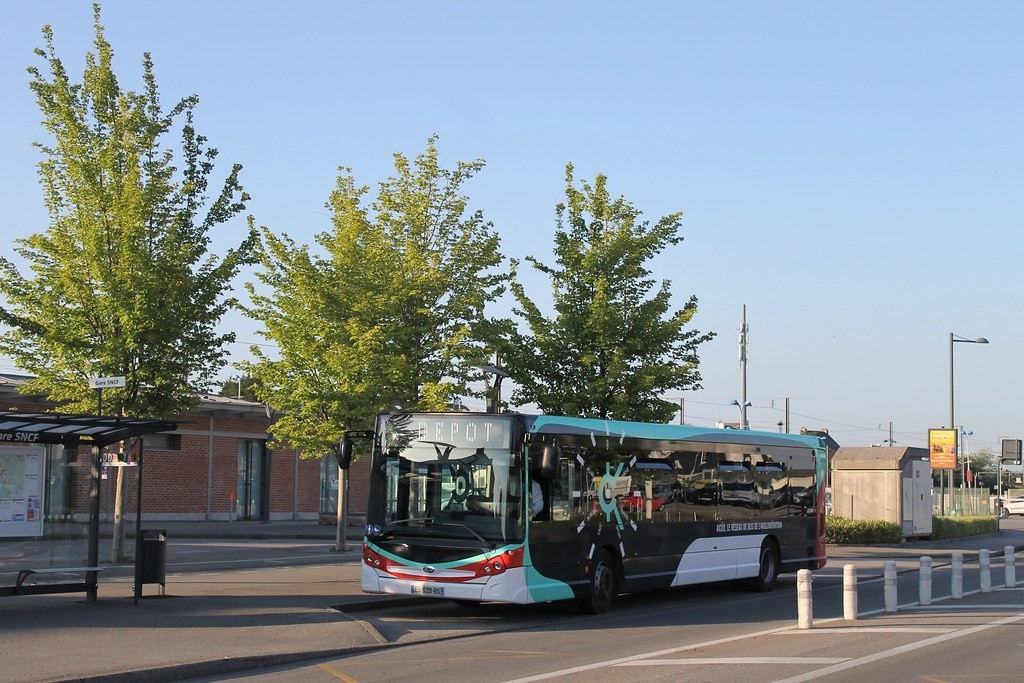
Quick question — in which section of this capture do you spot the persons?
[498,459,545,521]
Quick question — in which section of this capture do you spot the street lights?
[947,332,991,515]
[730,399,752,429]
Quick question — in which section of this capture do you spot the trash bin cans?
[140,530,167,596]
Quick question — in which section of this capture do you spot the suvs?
[1001,496,1024,517]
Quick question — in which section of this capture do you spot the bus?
[332,409,832,615]
